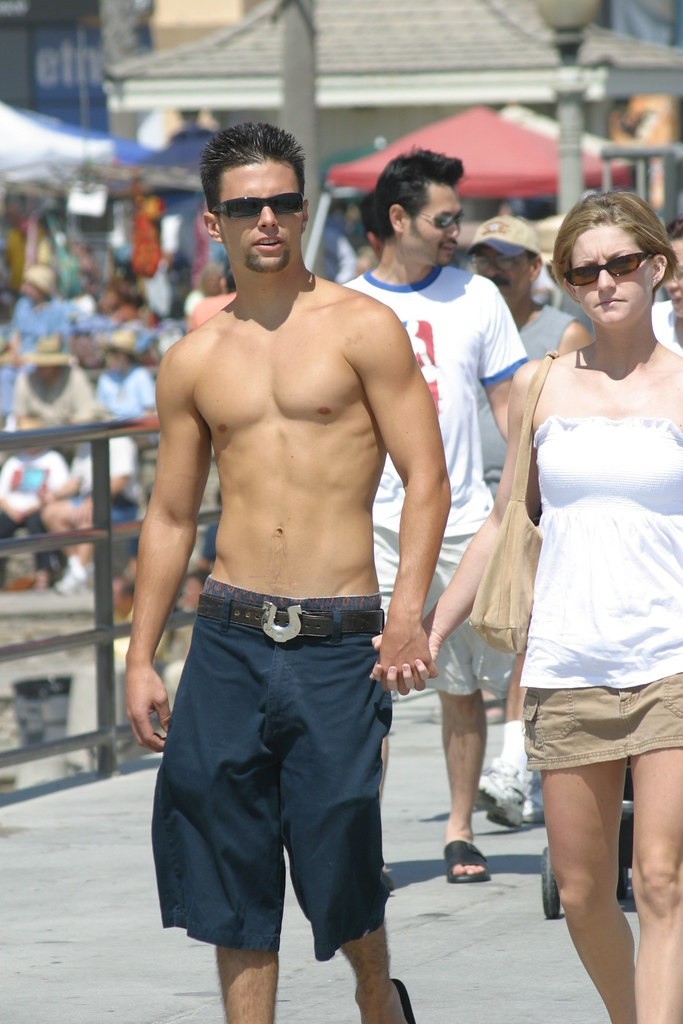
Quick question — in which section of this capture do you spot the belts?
[195,593,384,643]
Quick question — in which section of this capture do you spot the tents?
[329,107,632,203]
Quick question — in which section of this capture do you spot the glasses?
[563,250,656,286]
[403,203,464,227]
[210,192,304,219]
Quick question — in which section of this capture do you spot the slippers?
[381,871,394,891]
[390,979,415,1024]
[444,840,490,883]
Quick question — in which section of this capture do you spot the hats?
[19,335,69,367]
[26,266,60,301]
[94,328,140,356]
[466,214,540,256]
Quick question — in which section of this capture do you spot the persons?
[125,120,452,1024]
[0,265,184,605]
[183,260,237,334]
[369,191,683,1024]
[652,219,683,354]
[466,215,591,829]
[336,152,529,884]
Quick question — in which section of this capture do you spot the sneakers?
[476,758,531,828]
[523,769,545,823]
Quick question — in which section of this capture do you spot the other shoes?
[52,554,94,598]
[31,569,51,591]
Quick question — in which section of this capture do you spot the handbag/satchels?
[468,351,561,654]
